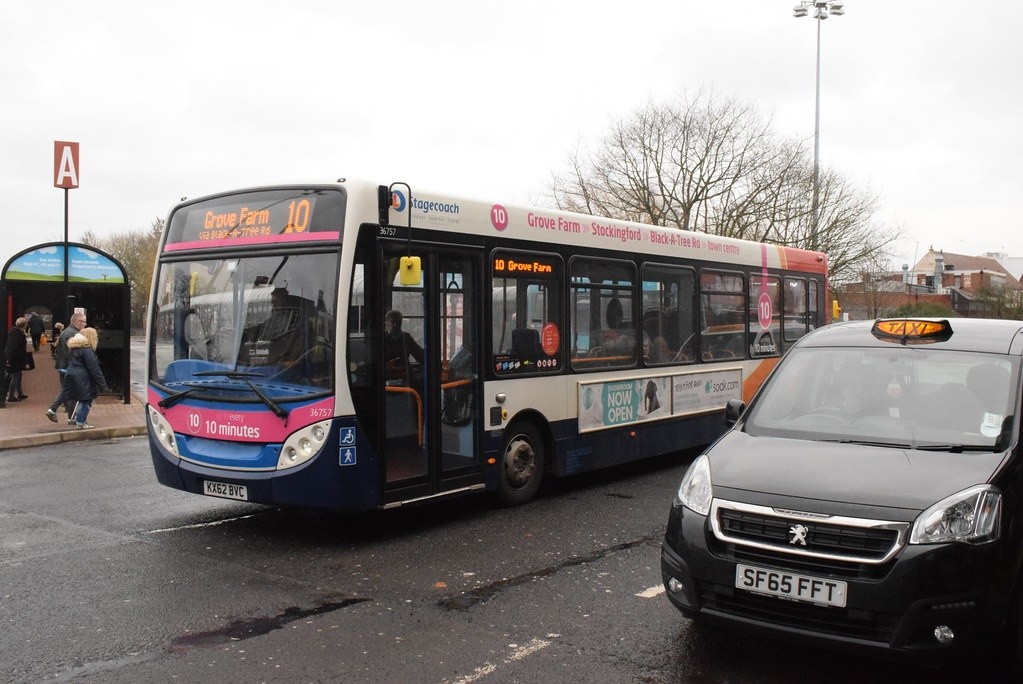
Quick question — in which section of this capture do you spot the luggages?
[0,368,11,408]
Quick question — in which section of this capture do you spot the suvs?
[660,317,1023,657]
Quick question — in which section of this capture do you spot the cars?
[672,321,816,363]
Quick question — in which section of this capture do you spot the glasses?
[77,320,86,324]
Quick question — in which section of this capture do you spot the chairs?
[511,327,542,359]
[836,362,1010,429]
[585,302,816,370]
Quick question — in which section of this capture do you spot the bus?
[145,178,842,509]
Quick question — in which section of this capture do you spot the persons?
[385,310,423,379]
[51,322,64,357]
[4,317,28,402]
[643,330,668,363]
[45,312,87,425]
[64,327,107,429]
[25,312,45,351]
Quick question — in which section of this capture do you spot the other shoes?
[68,417,76,425]
[76,421,95,429]
[8,396,21,402]
[18,394,28,399]
[46,409,58,423]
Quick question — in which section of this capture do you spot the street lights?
[792,0,845,251]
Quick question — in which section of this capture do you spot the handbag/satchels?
[40,333,47,345]
[441,384,475,427]
[21,352,36,370]
[64,373,71,383]
